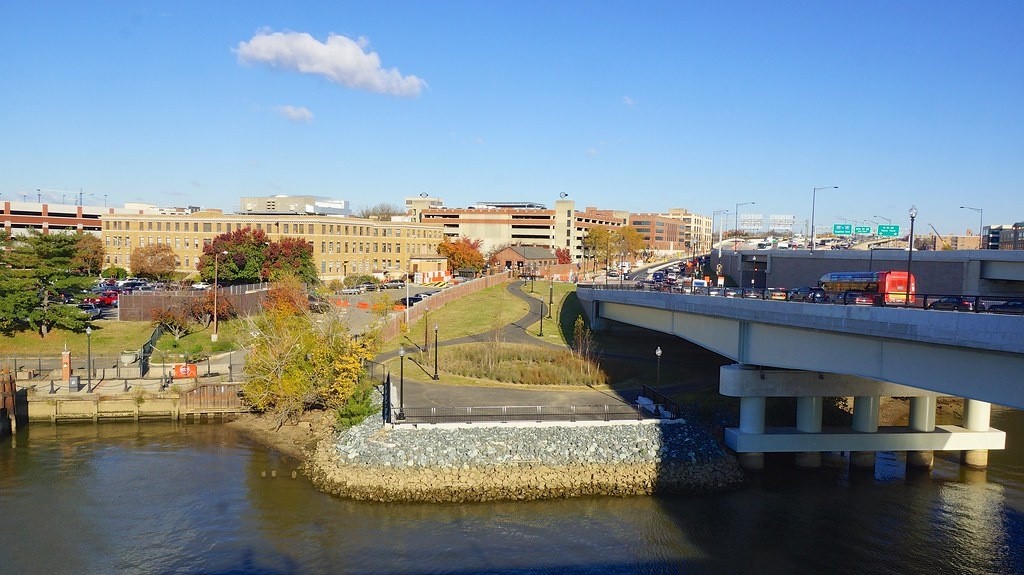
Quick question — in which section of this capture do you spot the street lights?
[811,186,839,249]
[423,306,430,352]
[902,204,918,308]
[86,326,93,394]
[432,323,440,380]
[751,255,757,293]
[873,215,892,248]
[620,252,625,289]
[734,201,756,252]
[711,209,729,250]
[388,266,410,332]
[692,238,698,273]
[652,346,664,419]
[959,206,983,250]
[864,219,879,244]
[397,347,407,420]
[211,250,229,341]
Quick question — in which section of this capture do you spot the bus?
[816,270,916,306]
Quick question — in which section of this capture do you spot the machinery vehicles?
[928,223,953,250]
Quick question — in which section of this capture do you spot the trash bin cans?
[69,376,80,392]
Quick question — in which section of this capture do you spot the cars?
[607,233,874,305]
[927,296,985,313]
[987,298,1024,315]
[38,277,229,321]
[306,279,442,314]
